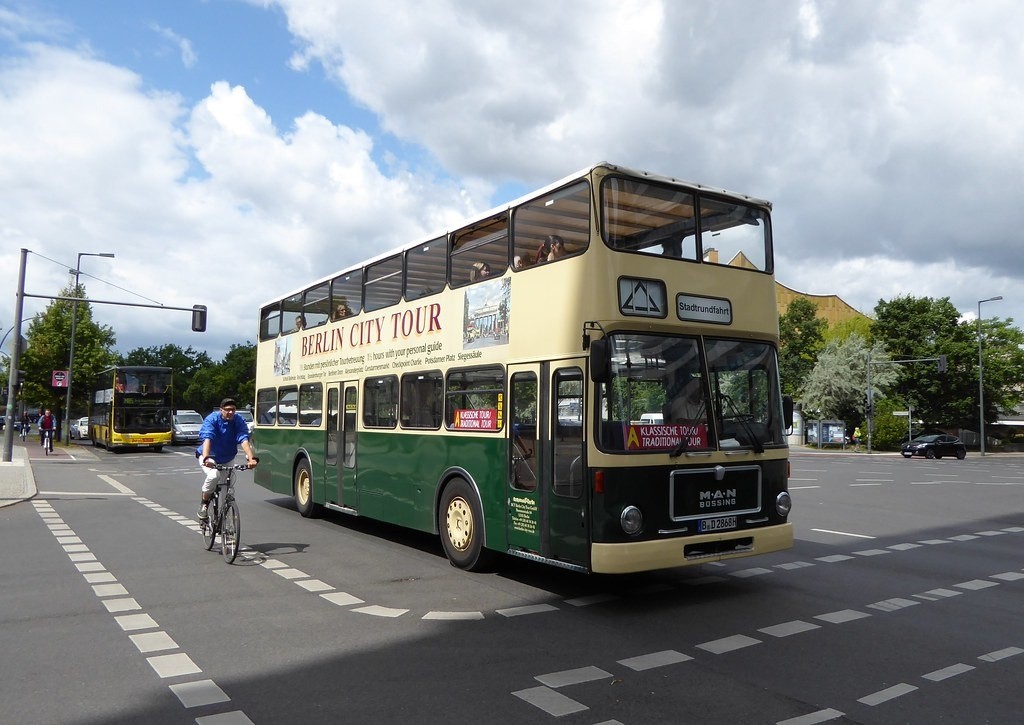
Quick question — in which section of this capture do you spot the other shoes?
[50,449,53,452]
[19,434,22,437]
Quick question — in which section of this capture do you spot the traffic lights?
[872,404,877,417]
[865,403,871,414]
[17,370,26,387]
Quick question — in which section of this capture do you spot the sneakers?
[197,498,210,519]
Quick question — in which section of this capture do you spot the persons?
[514,255,525,269]
[290,316,307,333]
[37,409,57,451]
[512,423,529,456]
[194,398,257,536]
[334,304,348,318]
[534,234,565,262]
[19,412,30,438]
[671,378,709,432]
[853,427,862,452]
[469,262,491,281]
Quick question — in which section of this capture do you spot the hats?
[220,398,238,408]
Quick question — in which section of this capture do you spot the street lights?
[65,252,114,444]
[978,295,1003,455]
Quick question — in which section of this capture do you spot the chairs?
[257,410,577,437]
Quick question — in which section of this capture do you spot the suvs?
[236,410,255,434]
[172,409,205,446]
[28,408,39,424]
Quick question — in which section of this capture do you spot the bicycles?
[19,422,32,442]
[197,454,260,564]
[39,429,56,456]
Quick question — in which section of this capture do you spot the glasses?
[223,406,236,411]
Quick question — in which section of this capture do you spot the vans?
[640,413,664,425]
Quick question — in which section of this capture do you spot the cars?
[70,416,91,440]
[0,416,21,431]
[901,434,967,460]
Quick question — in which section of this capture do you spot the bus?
[89,364,174,453]
[252,160,793,573]
